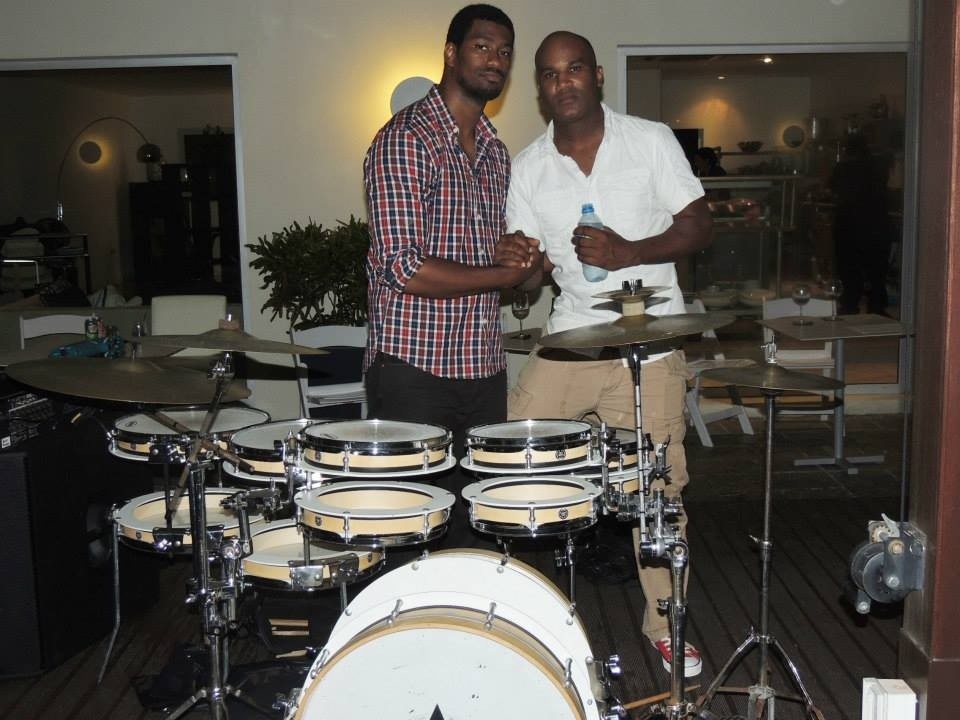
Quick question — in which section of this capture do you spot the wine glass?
[791,284,814,325]
[510,294,532,338]
[822,278,844,322]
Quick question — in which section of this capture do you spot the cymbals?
[535,311,739,351]
[591,285,673,303]
[3,355,253,404]
[700,363,848,398]
[120,313,333,357]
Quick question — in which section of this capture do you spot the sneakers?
[650,635,702,678]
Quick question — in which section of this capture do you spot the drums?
[287,547,626,720]
[105,400,664,663]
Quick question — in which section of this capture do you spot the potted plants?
[242,213,373,421]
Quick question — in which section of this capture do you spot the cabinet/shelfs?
[185,133,242,305]
[1,232,93,301]
[689,171,801,290]
[127,178,208,306]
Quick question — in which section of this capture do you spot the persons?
[360,1,543,447]
[502,32,713,676]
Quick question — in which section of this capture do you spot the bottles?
[577,203,610,282]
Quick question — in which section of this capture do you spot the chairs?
[152,295,228,359]
[289,325,371,425]
[19,311,97,353]
[761,295,847,444]
[680,305,756,447]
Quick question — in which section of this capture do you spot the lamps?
[79,141,101,164]
[56,116,163,223]
[782,126,805,149]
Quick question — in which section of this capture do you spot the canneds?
[83,315,144,350]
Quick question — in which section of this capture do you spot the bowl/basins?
[737,140,762,152]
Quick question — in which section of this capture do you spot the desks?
[757,312,914,478]
